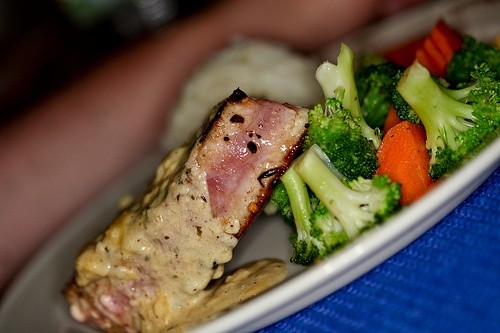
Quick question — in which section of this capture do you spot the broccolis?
[271,35,500,266]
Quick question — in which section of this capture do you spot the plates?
[0,0,500,333]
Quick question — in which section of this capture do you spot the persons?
[0,0,423,293]
